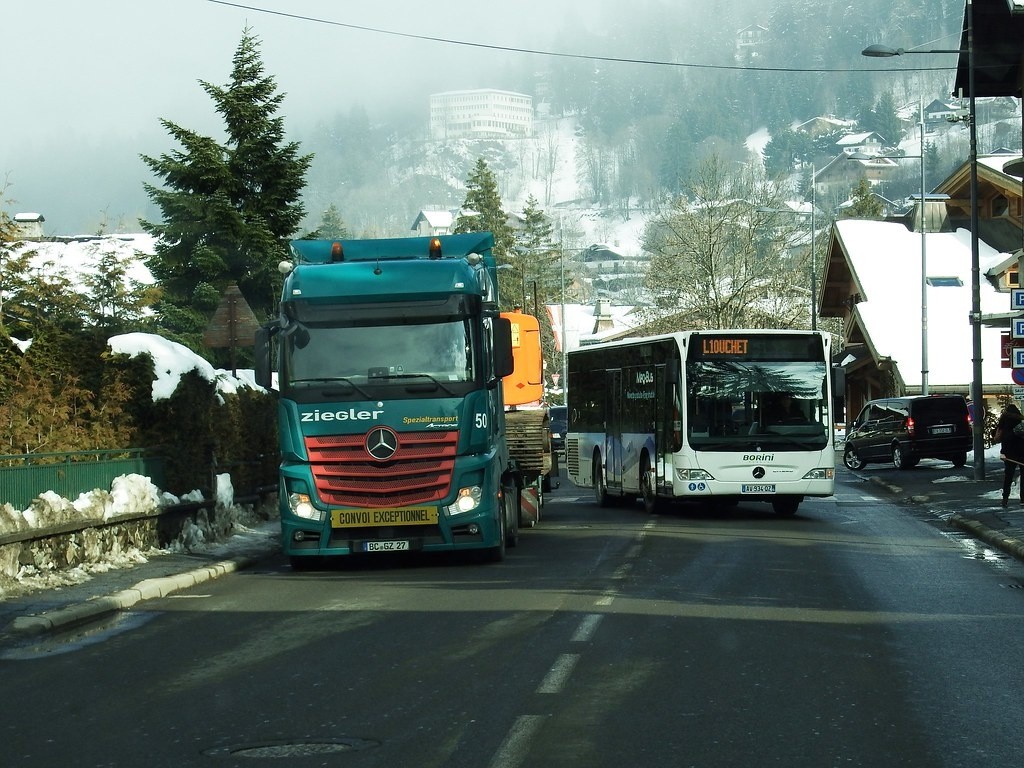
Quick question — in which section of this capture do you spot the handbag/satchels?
[992,426,1004,445]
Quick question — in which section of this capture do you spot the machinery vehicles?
[499,305,553,476]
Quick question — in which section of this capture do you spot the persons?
[992,403,1024,509]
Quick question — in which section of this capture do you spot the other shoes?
[1001,496,1009,508]
[1020,500,1024,505]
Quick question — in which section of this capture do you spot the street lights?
[862,1,985,483]
[756,164,816,329]
[846,95,928,396]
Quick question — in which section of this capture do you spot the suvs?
[547,406,567,448]
[842,396,973,471]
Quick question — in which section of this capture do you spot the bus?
[565,330,834,516]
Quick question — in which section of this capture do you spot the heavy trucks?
[254,233,560,571]
